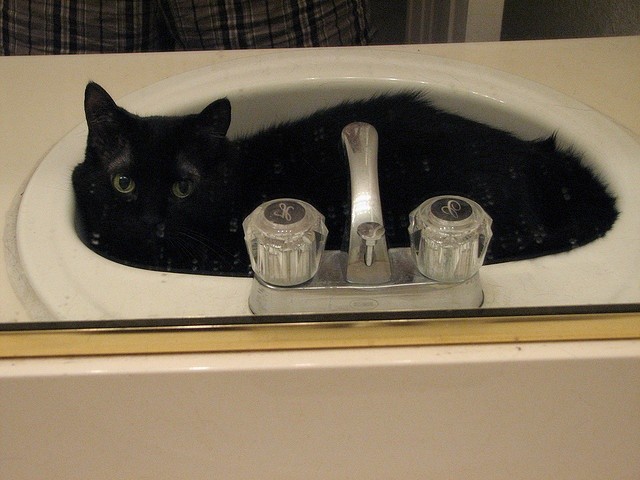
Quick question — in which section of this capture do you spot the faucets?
[339,120,391,286]
